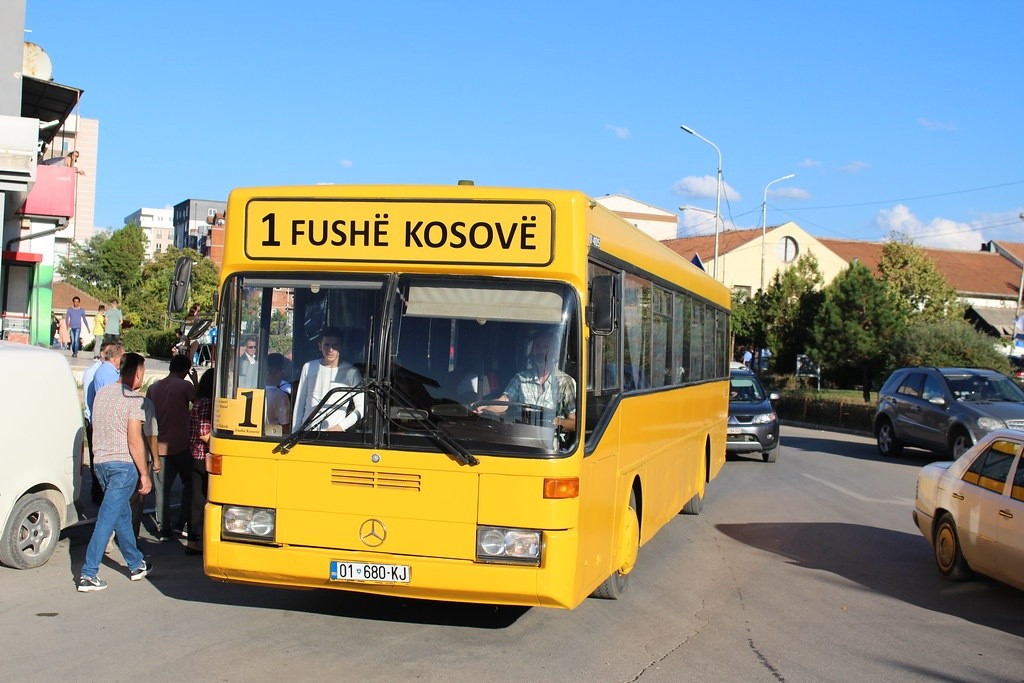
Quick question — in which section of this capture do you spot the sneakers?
[78,574,109,592]
[130,559,154,581]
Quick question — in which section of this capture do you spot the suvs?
[872,365,1024,462]
[726,366,781,462]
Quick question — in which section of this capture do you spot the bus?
[168,178,734,613]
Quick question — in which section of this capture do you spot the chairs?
[187,341,199,365]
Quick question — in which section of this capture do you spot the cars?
[913,427,1024,591]
[0,339,86,568]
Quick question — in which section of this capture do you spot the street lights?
[759,173,797,298]
[679,126,724,283]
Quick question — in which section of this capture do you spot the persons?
[50,296,123,361]
[77,342,215,592]
[41,151,85,176]
[470,331,576,451]
[731,387,746,399]
[172,303,201,367]
[739,347,759,369]
[238,337,292,434]
[291,326,368,434]
